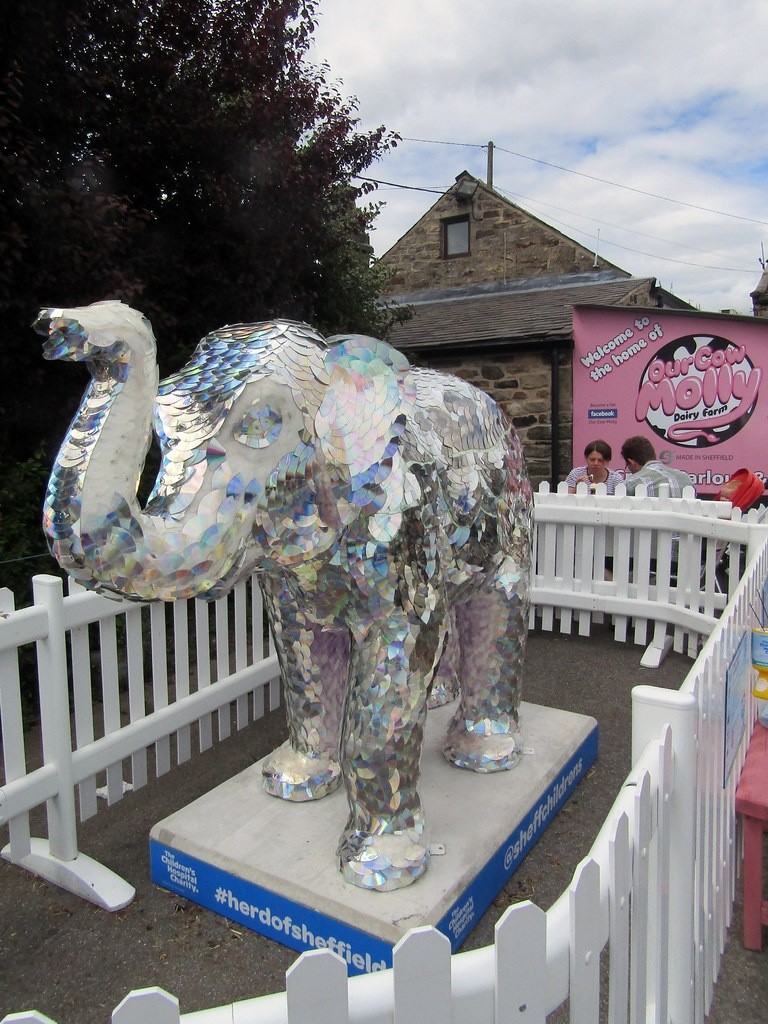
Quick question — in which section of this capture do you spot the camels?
[32,300,535,891]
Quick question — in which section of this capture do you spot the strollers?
[603,468,765,594]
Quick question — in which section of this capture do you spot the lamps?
[454,178,479,202]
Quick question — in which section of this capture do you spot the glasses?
[624,462,631,472]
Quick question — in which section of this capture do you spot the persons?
[564,439,624,570]
[719,479,743,503]
[615,436,697,597]
